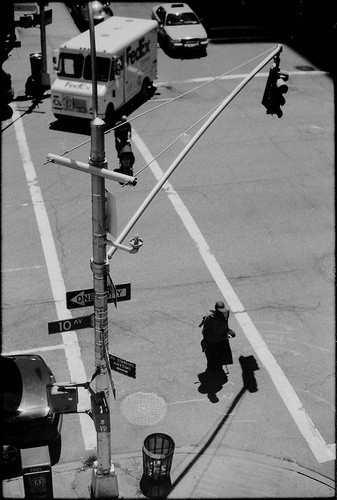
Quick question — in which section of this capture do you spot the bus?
[50,15,159,120]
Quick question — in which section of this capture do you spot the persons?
[202,300,236,367]
[114,116,133,160]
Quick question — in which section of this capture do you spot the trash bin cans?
[140,433,175,500]
[29,53,42,80]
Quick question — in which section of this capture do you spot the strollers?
[119,143,134,176]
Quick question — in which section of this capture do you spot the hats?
[215,302,229,313]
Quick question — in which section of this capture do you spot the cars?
[151,3,210,52]
[0,354,62,445]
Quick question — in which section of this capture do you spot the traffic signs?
[65,283,130,309]
[106,353,135,379]
[48,314,94,336]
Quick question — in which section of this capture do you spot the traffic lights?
[261,55,289,117]
